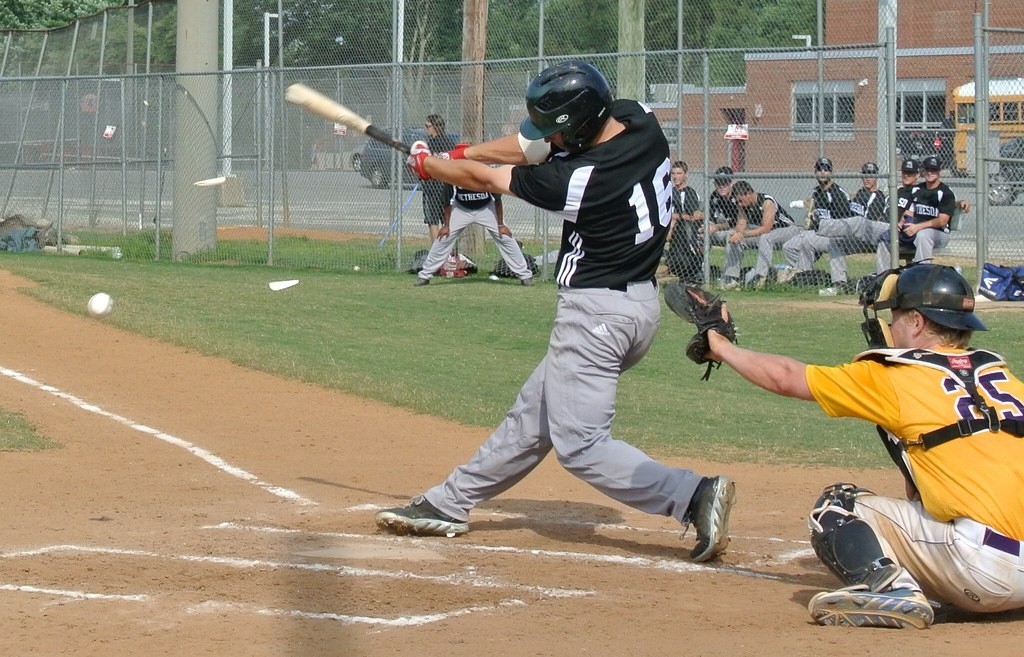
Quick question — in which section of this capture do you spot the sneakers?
[520,275,535,287]
[807,583,933,630]
[375,493,469,538]
[414,277,430,287]
[679,474,737,564]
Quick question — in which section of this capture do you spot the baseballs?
[87,293,113,317]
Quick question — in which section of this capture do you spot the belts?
[946,518,1021,557]
[608,275,658,293]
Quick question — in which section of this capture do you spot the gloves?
[405,140,433,181]
[433,143,470,160]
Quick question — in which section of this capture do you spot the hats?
[813,157,832,172]
[900,159,920,173]
[860,162,879,174]
[921,157,941,170]
[713,166,734,186]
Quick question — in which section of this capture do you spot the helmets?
[893,264,989,331]
[519,60,615,149]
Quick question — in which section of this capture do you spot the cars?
[987,138,1024,207]
[351,142,367,172]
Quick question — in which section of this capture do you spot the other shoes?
[746,276,767,291]
[717,277,742,293]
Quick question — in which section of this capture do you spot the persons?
[664,263,1024,629]
[666,161,797,289]
[374,62,737,564]
[415,144,535,286]
[421,114,452,243]
[782,158,885,275]
[803,159,969,281]
[876,157,955,275]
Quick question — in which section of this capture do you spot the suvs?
[360,128,458,188]
[934,116,974,177]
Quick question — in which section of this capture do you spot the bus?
[952,75,1024,175]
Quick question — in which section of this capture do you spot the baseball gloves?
[663,278,736,365]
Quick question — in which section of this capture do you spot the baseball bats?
[284,82,411,155]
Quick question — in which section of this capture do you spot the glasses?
[425,123,433,129]
[816,164,831,171]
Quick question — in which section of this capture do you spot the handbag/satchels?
[979,263,1024,301]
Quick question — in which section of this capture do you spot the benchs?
[752,204,965,263]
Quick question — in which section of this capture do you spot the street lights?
[264,12,278,170]
